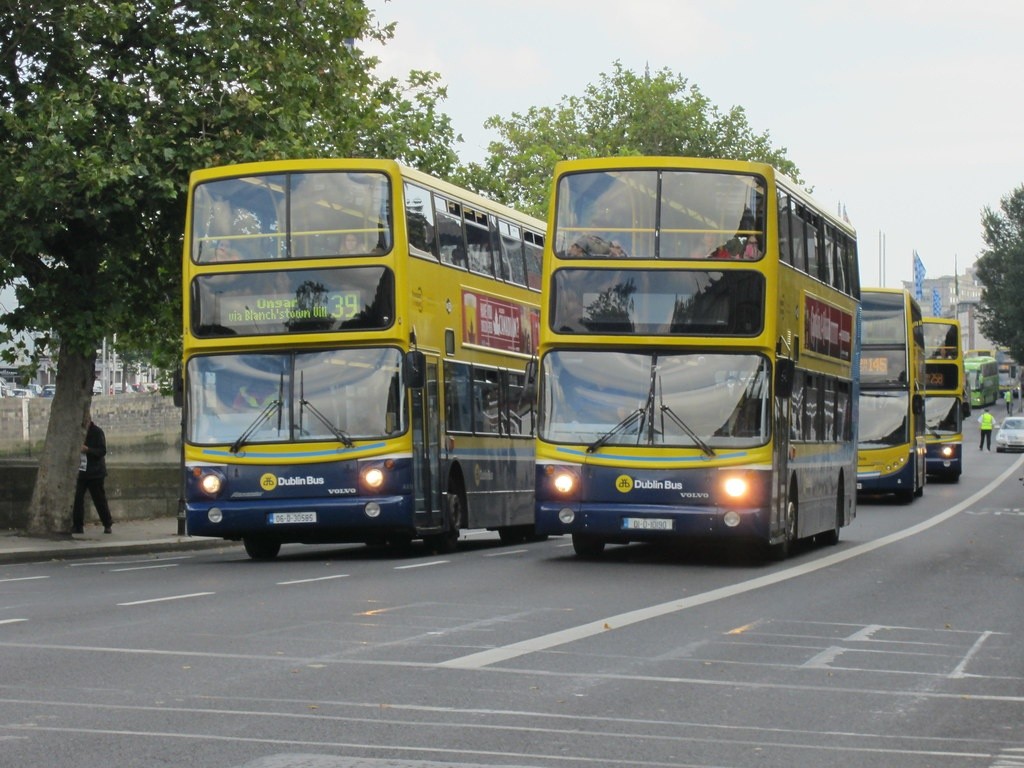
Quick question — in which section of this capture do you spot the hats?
[984,408,989,411]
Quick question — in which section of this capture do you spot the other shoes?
[988,448,990,451]
[980,447,983,450]
[104,525,110,534]
[70,524,83,534]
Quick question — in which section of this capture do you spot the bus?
[863,317,972,483]
[963,356,999,406]
[860,288,927,502]
[524,155,862,563]
[967,349,994,358]
[994,347,1024,398]
[172,159,584,561]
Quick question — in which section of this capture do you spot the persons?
[977,408,996,451]
[1004,389,1011,414]
[570,221,723,261]
[340,234,360,254]
[68,421,113,534]
[209,239,239,262]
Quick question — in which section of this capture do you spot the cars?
[110,383,131,393]
[5,389,34,397]
[92,381,102,393]
[40,385,56,398]
[26,384,42,394]
[995,417,1024,452]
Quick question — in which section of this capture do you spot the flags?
[933,289,942,317]
[838,202,841,217]
[914,254,925,301]
[843,205,851,224]
